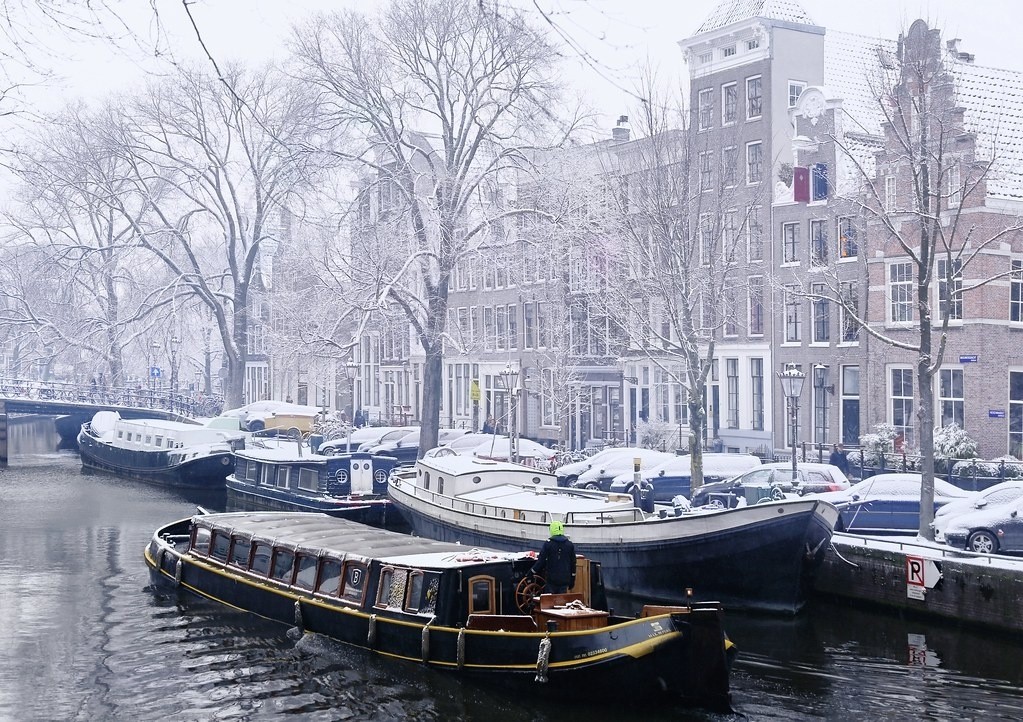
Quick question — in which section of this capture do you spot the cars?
[935,481,1022,553]
[553,446,764,503]
[244,411,320,440]
[318,425,565,468]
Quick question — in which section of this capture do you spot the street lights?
[168,336,182,412]
[499,364,520,461]
[774,362,805,495]
[150,340,160,404]
[342,356,358,430]
[194,369,202,390]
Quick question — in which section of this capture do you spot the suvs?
[691,464,851,510]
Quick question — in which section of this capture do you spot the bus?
[801,474,979,535]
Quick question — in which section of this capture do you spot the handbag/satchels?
[547,561,570,586]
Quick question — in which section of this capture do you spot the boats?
[142,510,740,715]
[77,412,245,489]
[223,450,399,525]
[386,458,842,614]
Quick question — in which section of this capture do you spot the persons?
[527,520,576,594]
[829,443,851,478]
[482,414,499,435]
[88,374,97,404]
[97,372,113,405]
[354,409,366,428]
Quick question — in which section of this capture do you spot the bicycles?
[200,392,225,418]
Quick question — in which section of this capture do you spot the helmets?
[549,521,564,535]
